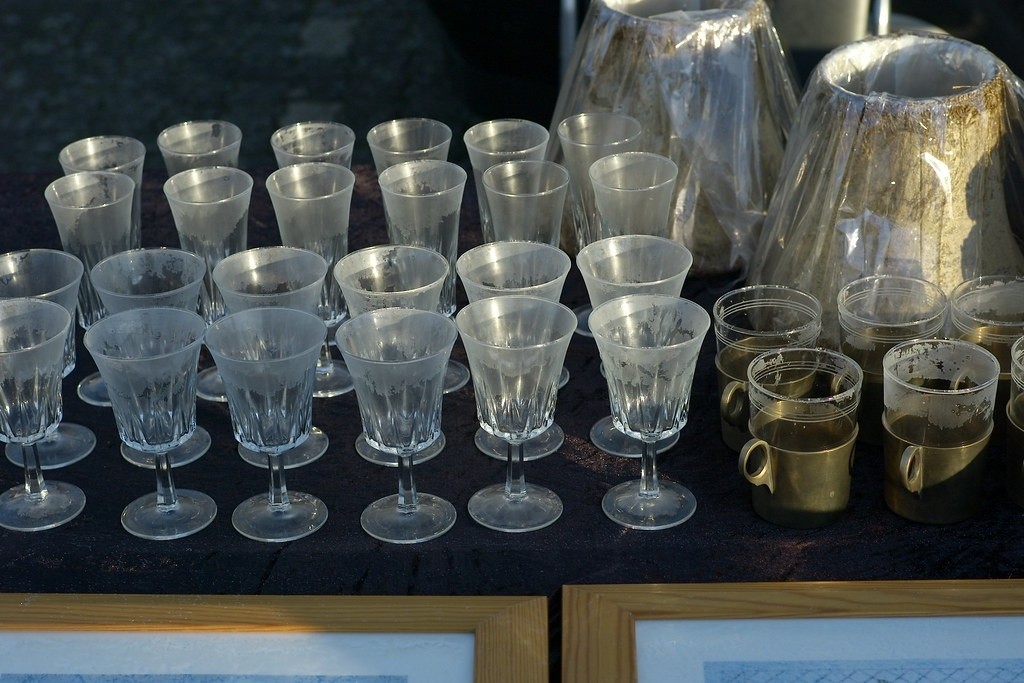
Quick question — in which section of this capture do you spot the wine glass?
[0,299,85,532]
[575,234,693,458]
[335,309,459,543]
[204,306,330,542]
[83,307,218,540]
[0,249,84,469]
[456,295,578,533]
[333,243,450,467]
[211,246,333,469]
[588,295,711,530]
[89,248,206,469]
[455,240,571,461]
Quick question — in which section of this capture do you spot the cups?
[44,114,680,408]
[713,275,1024,513]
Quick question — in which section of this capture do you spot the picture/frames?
[563,582,1024,683]
[0,597,548,683]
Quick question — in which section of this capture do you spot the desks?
[0,0,1024,588]
